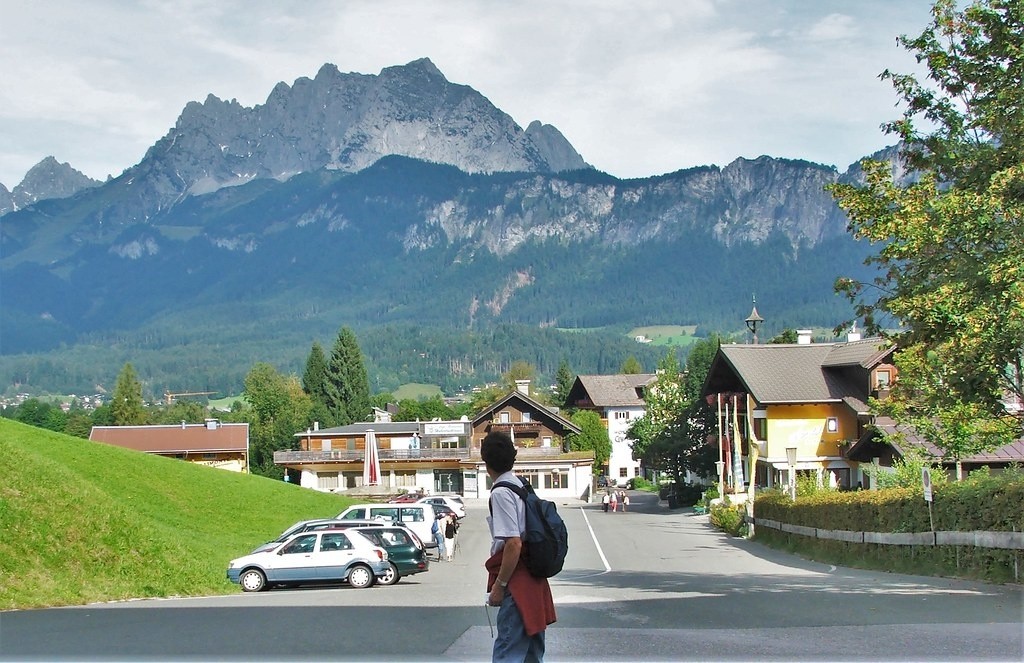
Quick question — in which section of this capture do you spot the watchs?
[496,577,508,587]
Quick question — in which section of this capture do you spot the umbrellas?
[363,429,382,486]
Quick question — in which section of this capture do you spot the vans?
[333,504,438,549]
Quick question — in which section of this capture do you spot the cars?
[598,475,608,487]
[384,493,467,531]
[306,525,429,587]
[226,529,392,591]
[249,519,408,553]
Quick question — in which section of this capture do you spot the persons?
[480,431,558,663]
[601,491,610,512]
[610,489,619,513]
[620,491,627,512]
[433,511,460,561]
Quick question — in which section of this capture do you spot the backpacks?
[488,476,568,579]
[431,520,438,534]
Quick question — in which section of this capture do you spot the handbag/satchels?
[602,506,604,510]
